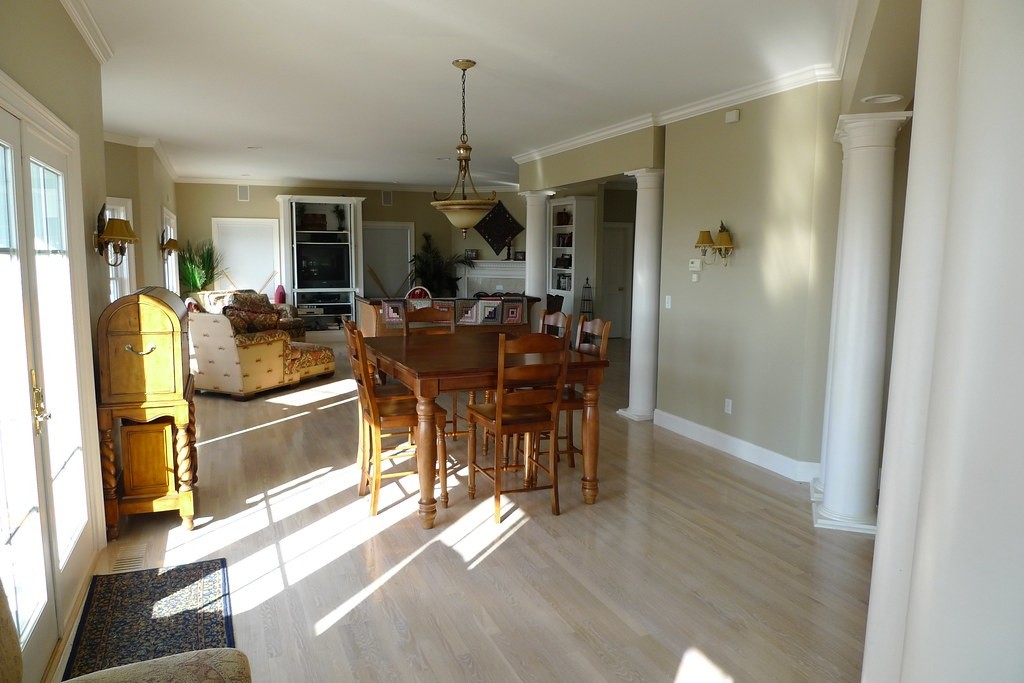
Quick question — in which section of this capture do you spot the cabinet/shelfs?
[98,374,198,541]
[546,195,597,351]
[275,195,366,331]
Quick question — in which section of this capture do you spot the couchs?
[187,312,335,401]
[205,289,305,343]
[1,578,252,683]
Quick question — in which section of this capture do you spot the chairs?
[344,325,449,516]
[513,314,612,492]
[483,309,572,470]
[341,315,419,475]
[403,308,470,446]
[465,332,572,524]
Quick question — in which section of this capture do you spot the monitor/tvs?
[296,243,350,288]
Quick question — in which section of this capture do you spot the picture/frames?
[514,252,525,261]
[464,249,478,260]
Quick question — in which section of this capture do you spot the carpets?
[60,558,235,681]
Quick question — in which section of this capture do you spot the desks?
[364,332,609,530]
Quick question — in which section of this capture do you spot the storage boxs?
[98,286,190,403]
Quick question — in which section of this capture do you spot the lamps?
[694,231,734,266]
[94,218,139,267]
[430,59,498,240]
[160,238,179,260]
